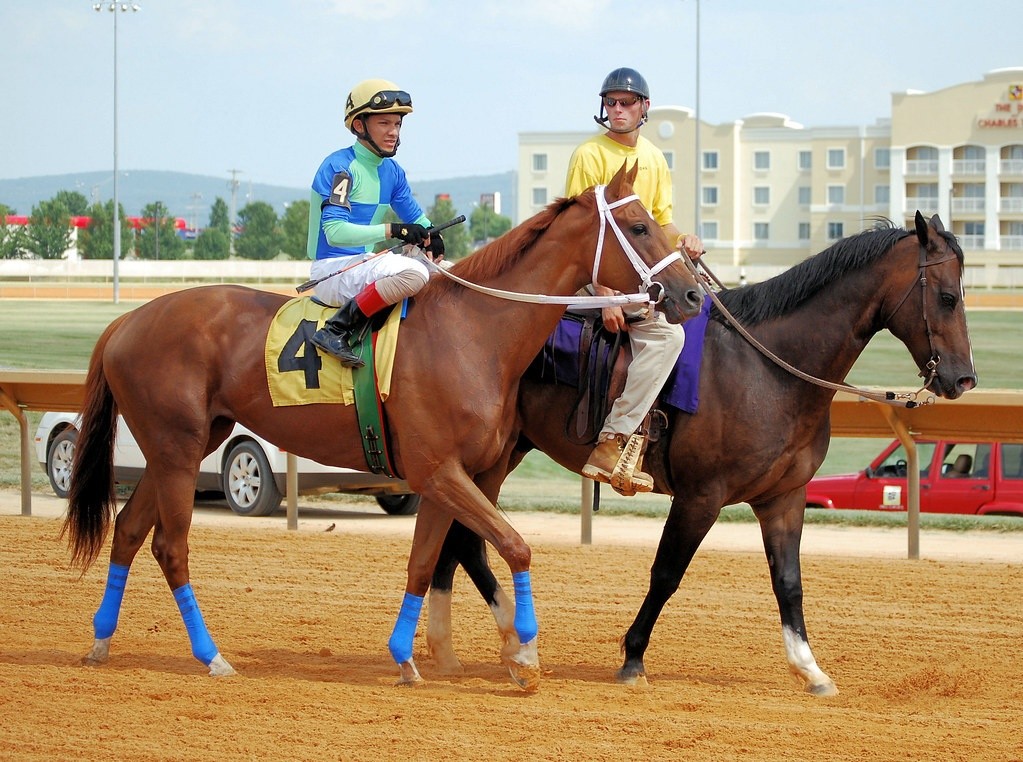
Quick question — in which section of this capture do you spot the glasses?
[602,95,641,107]
[368,90,413,109]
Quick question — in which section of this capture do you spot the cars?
[34,410,422,518]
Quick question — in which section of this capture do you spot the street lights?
[92,0,142,302]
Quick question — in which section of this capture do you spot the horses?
[427,212,977,696]
[59,156,702,692]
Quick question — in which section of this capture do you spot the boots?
[583,435,655,494]
[310,298,368,368]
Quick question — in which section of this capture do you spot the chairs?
[948,454,972,478]
[973,453,989,477]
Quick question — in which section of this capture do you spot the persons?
[566,67,703,492]
[307,79,455,369]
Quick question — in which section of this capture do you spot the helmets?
[344,79,414,130]
[599,67,649,101]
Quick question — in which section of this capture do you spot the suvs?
[806,435,1023,517]
